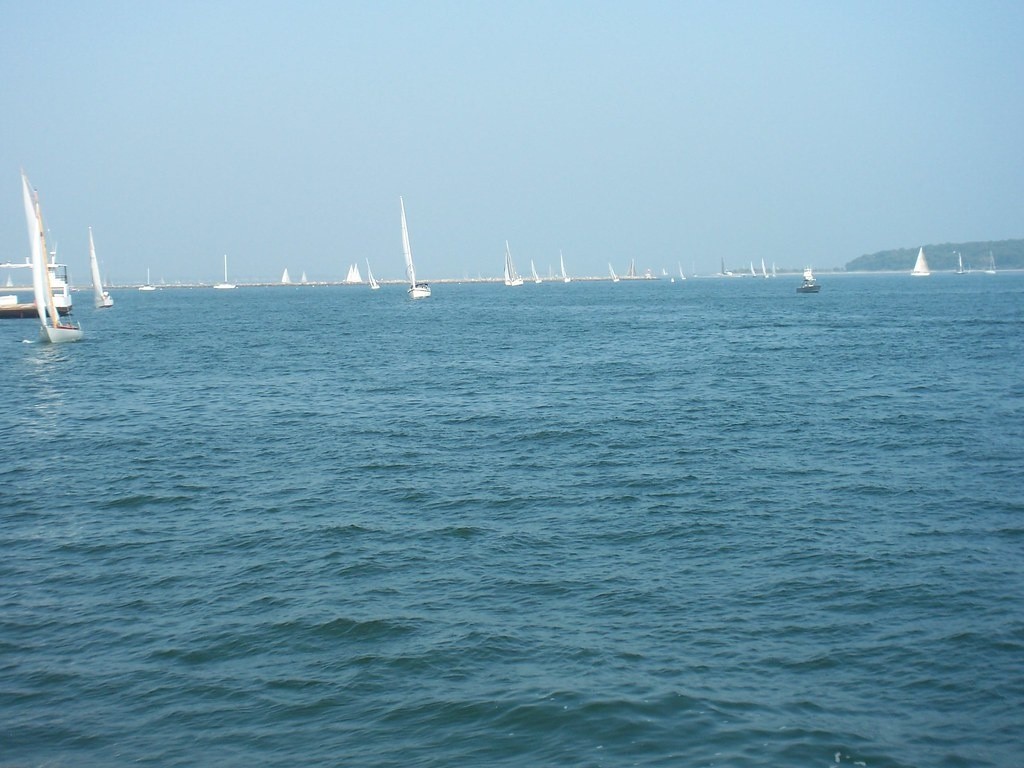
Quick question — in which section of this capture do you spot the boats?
[139,254,236,290]
[0,243,72,317]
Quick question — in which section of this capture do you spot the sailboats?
[796,269,821,293]
[88,226,114,308]
[529,253,776,284]
[504,239,523,286]
[282,258,380,290]
[955,251,998,274]
[400,195,432,298]
[911,246,930,276]
[20,167,83,342]
